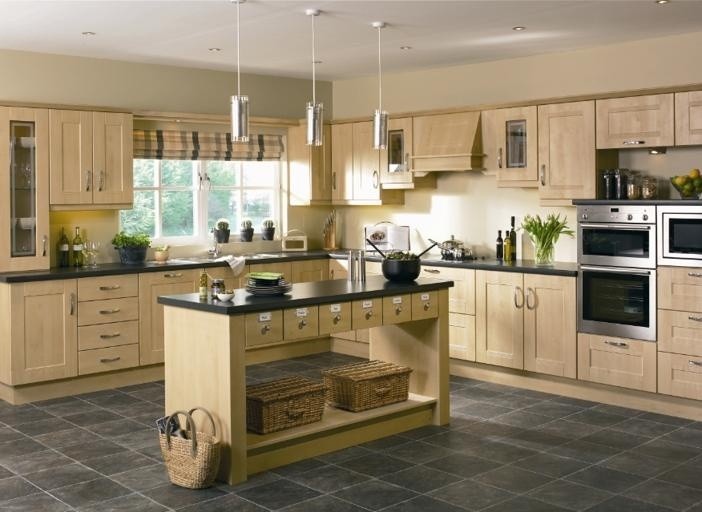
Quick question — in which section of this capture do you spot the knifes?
[321,209,336,234]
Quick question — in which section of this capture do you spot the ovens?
[577,204,657,341]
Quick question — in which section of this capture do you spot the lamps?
[305,8,324,147]
[373,20,388,151]
[229,0,250,143]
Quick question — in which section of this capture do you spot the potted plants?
[213,218,230,243]
[261,218,275,240]
[111,229,151,266]
[241,220,254,242]
[515,208,575,267]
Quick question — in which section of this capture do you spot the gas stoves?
[423,255,489,263]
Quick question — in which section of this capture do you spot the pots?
[438,246,473,259]
[381,259,421,281]
[428,235,464,257]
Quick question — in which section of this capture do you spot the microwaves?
[654,206,702,267]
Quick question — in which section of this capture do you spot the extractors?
[409,110,486,172]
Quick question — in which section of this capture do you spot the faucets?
[210,227,223,258]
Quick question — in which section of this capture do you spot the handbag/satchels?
[159,407,221,489]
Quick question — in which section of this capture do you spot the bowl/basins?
[669,176,702,199]
[18,137,35,148]
[155,251,169,264]
[217,293,235,301]
[18,217,35,229]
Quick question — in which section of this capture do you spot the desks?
[157,277,454,486]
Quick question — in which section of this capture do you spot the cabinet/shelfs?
[245,259,330,286]
[540,97,596,209]
[494,105,540,189]
[596,91,702,149]
[576,331,657,395]
[333,121,405,205]
[48,108,133,210]
[329,258,382,344]
[475,269,577,380]
[0,104,50,272]
[138,266,240,366]
[382,114,439,190]
[657,267,701,401]
[78,273,139,376]
[288,126,332,207]
[0,279,77,387]
[418,266,476,362]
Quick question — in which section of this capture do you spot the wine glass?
[83,240,92,270]
[91,241,104,265]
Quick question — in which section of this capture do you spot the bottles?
[59,226,69,266]
[599,167,657,199]
[10,159,31,186]
[358,251,366,282]
[496,216,516,261]
[72,226,85,268]
[347,250,356,281]
[199,267,207,299]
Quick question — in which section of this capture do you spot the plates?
[369,230,384,241]
[245,272,294,296]
